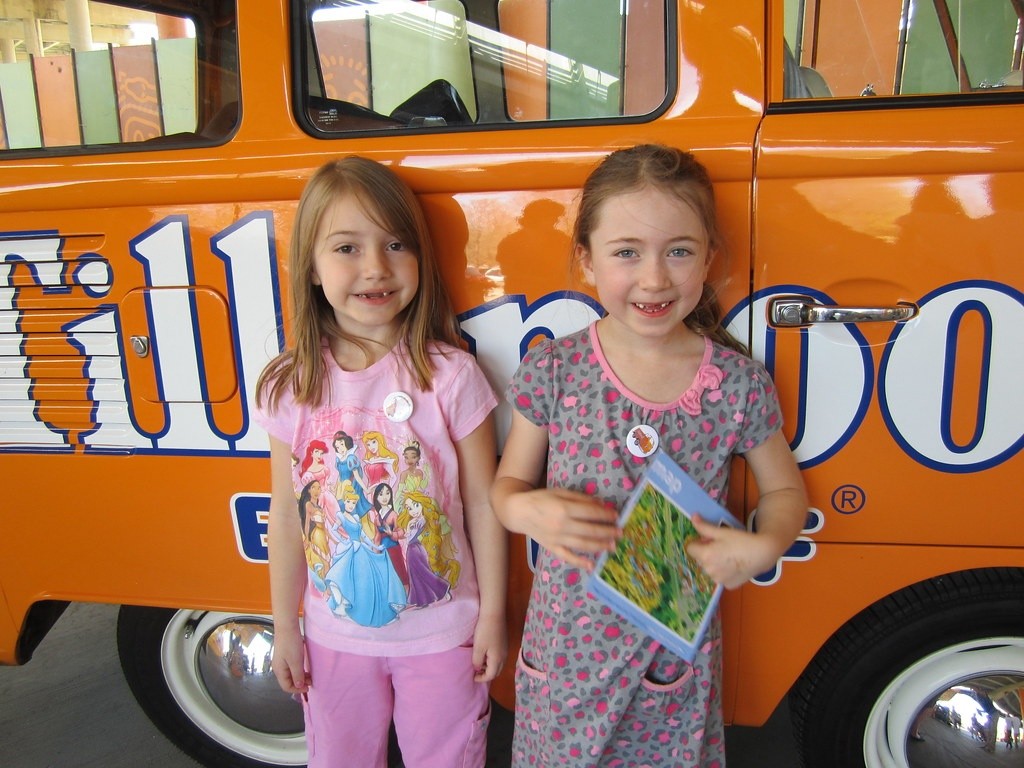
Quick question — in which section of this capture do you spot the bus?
[0,1,1023,768]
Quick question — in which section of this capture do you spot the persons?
[253,156,507,768]
[489,145,808,767]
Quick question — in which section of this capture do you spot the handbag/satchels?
[1004,728,1009,742]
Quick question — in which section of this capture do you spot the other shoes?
[909,732,926,742]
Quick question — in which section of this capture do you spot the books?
[590,452,747,664]
